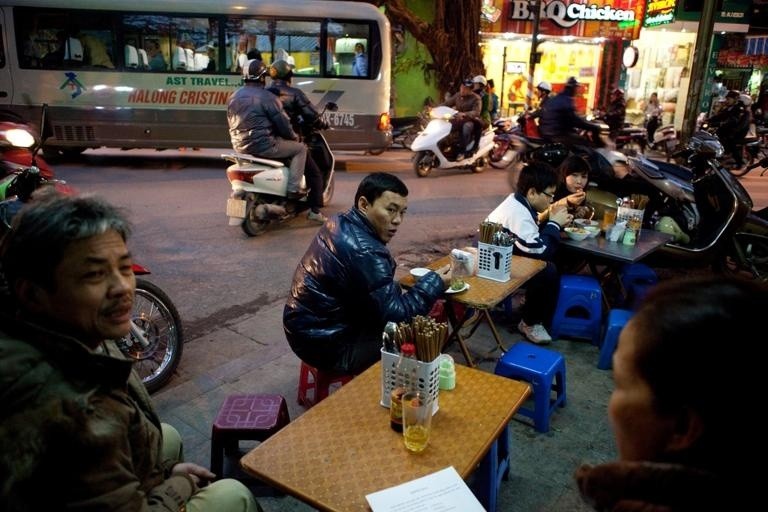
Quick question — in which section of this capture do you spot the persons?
[426,71,768,352]
[282,171,454,376]
[351,42,369,76]
[575,262,768,511]
[226,58,311,200]
[31,35,295,76]
[264,60,331,223]
[0,192,264,512]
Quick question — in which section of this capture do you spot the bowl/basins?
[547,192,651,248]
[409,266,432,282]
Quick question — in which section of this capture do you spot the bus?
[0,0,393,156]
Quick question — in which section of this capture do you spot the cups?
[401,389,434,454]
[448,255,475,278]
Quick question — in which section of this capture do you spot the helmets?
[537,81,551,93]
[725,90,740,99]
[612,88,624,98]
[472,75,487,87]
[270,60,293,80]
[242,59,268,81]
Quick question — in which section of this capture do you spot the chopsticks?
[477,220,503,272]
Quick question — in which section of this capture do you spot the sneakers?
[307,211,328,222]
[501,292,526,310]
[517,318,552,344]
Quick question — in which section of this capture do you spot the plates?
[443,280,468,293]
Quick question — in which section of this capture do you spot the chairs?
[63,34,295,75]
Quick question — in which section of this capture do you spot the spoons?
[491,229,518,276]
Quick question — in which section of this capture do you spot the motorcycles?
[219,102,340,238]
[0,102,183,395]
[0,113,78,202]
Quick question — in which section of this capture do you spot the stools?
[463,424,510,512]
[493,340,567,433]
[621,264,657,304]
[297,361,353,411]
[552,275,602,345]
[597,308,637,369]
[211,392,290,499]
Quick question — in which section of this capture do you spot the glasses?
[542,191,555,199]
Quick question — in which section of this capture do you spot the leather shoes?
[288,187,307,196]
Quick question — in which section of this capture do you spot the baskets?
[615,206,645,241]
[380,346,442,417]
[475,240,514,283]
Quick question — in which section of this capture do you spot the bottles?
[387,343,420,432]
